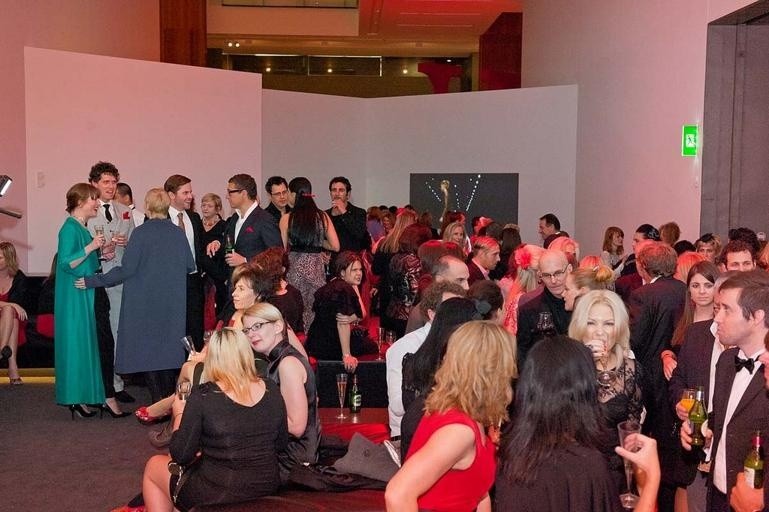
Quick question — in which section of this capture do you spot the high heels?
[136,406,172,427]
[7,371,24,385]
[69,403,97,421]
[100,401,132,419]
[148,429,173,450]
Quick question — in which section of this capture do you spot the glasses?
[538,266,568,278]
[272,190,289,196]
[241,321,275,335]
[227,189,241,195]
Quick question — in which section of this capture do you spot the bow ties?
[734,355,755,374]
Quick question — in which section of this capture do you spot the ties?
[102,204,113,222]
[177,213,185,233]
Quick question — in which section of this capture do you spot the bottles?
[744,431,764,490]
[350,375,362,412]
[688,386,706,446]
[224,234,233,254]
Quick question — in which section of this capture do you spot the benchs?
[191,407,393,512]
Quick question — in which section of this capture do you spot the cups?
[332,196,341,208]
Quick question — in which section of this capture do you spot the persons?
[0,242,29,389]
[52,157,769,512]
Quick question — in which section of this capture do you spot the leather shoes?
[115,390,135,403]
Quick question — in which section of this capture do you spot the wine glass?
[536,312,555,333]
[387,332,395,346]
[181,335,196,358]
[178,383,191,400]
[617,421,642,509]
[337,374,347,420]
[203,331,211,342]
[375,328,385,361]
[94,224,106,260]
[682,389,696,413]
[592,331,617,386]
